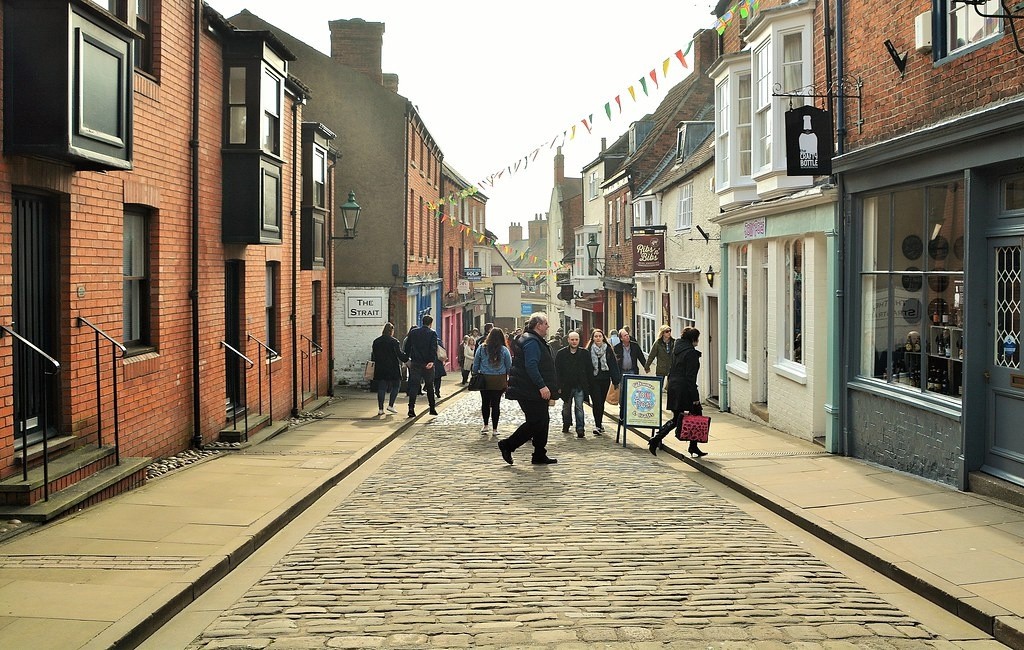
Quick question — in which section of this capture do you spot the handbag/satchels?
[364,360,375,380]
[675,404,711,443]
[606,381,620,405]
[436,340,447,362]
[468,373,485,391]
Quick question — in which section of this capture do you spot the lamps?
[472,286,493,306]
[705,265,714,287]
[586,233,605,270]
[331,189,362,240]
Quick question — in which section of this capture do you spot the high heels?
[689,446,708,455]
[648,437,658,456]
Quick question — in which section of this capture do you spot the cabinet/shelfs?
[904,325,963,395]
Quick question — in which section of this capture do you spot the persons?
[874,330,921,376]
[786,333,801,364]
[405,315,438,417]
[370,322,407,416]
[498,311,558,464]
[402,323,675,437]
[648,326,709,457]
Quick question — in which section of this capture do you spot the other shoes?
[387,406,398,413]
[498,439,513,464]
[600,425,605,432]
[531,453,557,464]
[408,411,416,417]
[577,430,584,438]
[481,427,489,432]
[378,410,384,414]
[435,392,441,398]
[430,410,438,416]
[562,424,570,432]
[492,431,501,435]
[417,391,424,395]
[592,428,602,435]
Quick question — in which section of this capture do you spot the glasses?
[666,329,671,333]
